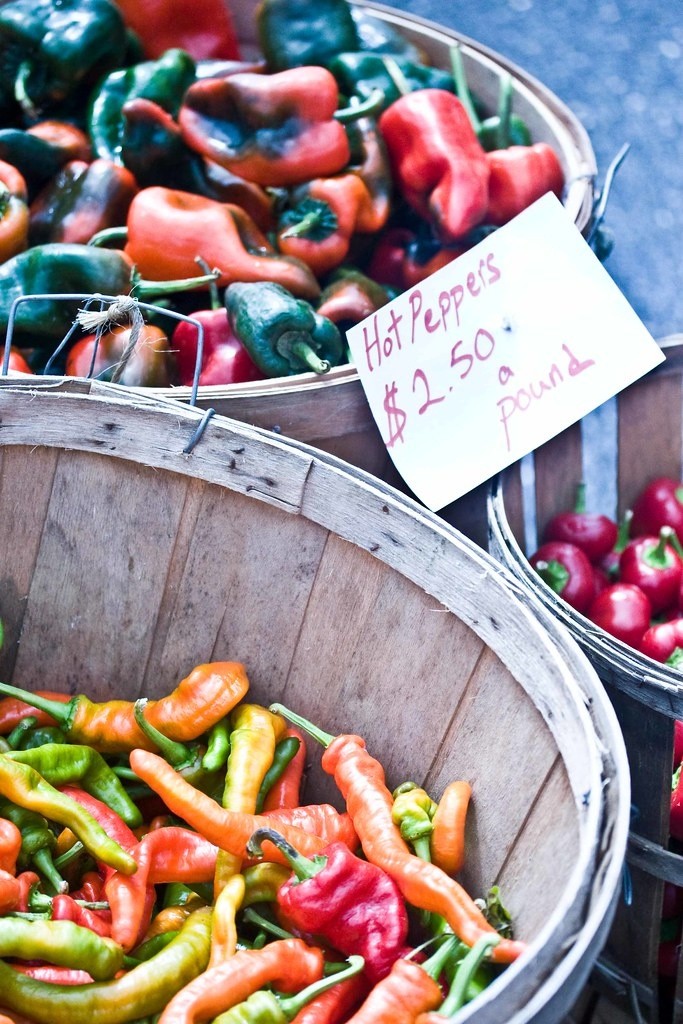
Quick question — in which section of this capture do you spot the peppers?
[529,477,682,975]
[0,0,565,386]
[0,663,523,1024]
